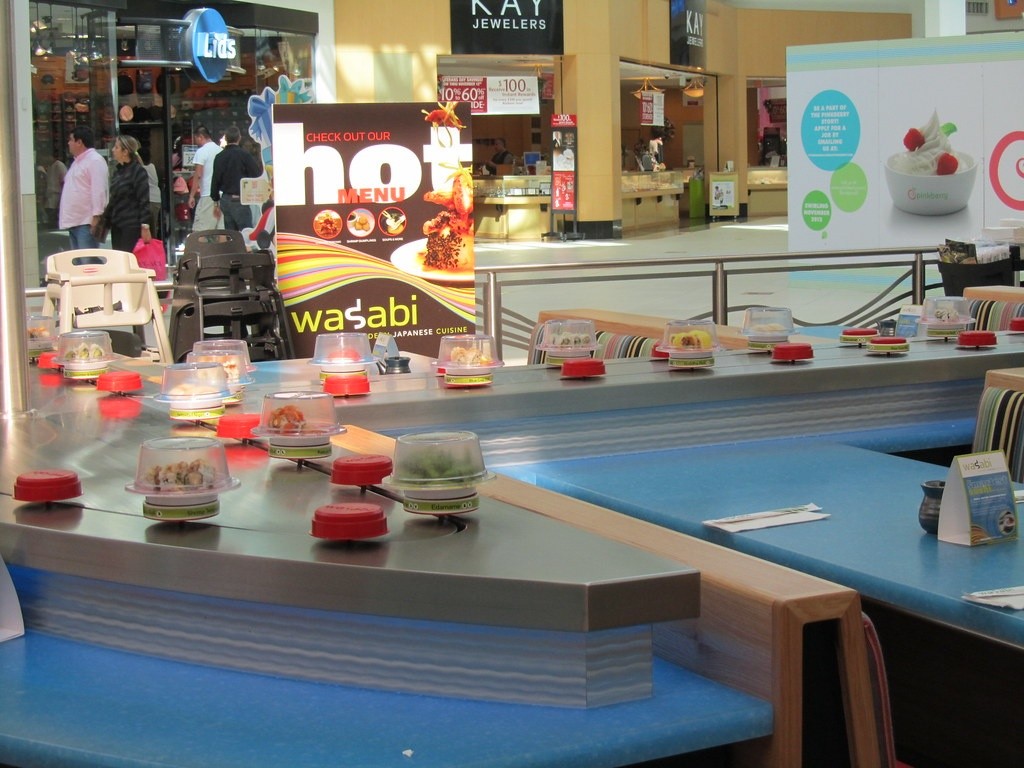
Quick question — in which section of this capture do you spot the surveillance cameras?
[686,78,692,82]
[664,76,670,79]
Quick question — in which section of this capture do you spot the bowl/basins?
[536,319,602,352]
[186,349,254,386]
[192,340,255,373]
[382,430,498,489]
[430,334,505,369]
[124,436,241,496]
[655,320,726,353]
[152,361,236,404]
[55,331,117,366]
[738,307,799,336]
[26,315,57,342]
[915,296,976,325]
[250,391,347,438]
[884,149,978,216]
[308,332,378,367]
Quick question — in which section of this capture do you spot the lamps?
[680,79,705,97]
[632,79,665,101]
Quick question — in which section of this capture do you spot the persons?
[485,138,513,175]
[44,151,67,228]
[58,125,260,266]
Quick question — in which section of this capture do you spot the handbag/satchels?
[133,238,168,281]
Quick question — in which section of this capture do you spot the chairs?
[42,229,298,361]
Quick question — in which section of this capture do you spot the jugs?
[376,356,411,375]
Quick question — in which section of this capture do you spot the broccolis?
[391,448,483,491]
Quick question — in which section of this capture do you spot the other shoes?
[161,292,171,313]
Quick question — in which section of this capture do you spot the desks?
[1,637,781,768]
[531,446,1023,650]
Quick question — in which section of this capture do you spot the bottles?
[918,480,946,535]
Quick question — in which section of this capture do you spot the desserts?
[269,405,311,434]
[27,324,106,362]
[421,169,473,270]
[165,337,249,397]
[322,324,798,387]
[142,457,220,493]
[886,112,977,215]
[936,307,960,323]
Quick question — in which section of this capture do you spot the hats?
[173,177,202,195]
[177,204,191,222]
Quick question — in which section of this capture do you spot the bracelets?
[214,203,219,207]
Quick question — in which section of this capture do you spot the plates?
[390,238,475,283]
